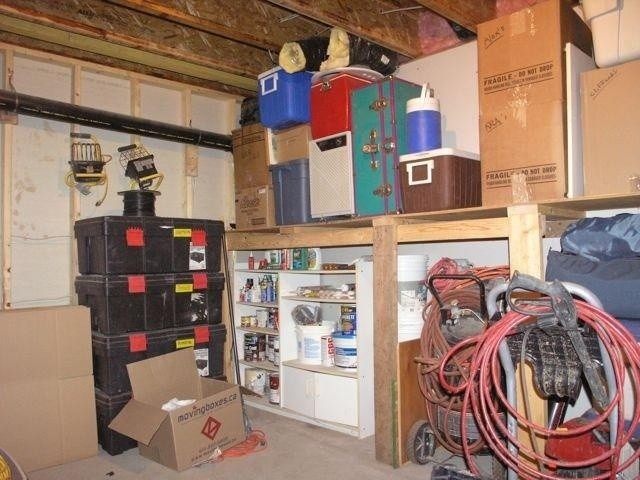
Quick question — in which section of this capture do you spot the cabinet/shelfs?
[225,246,373,442]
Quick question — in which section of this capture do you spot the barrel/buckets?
[331,331,357,367]
[295,321,335,365]
[406,82,441,154]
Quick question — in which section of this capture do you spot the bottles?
[249,251,254,270]
[240,273,278,304]
